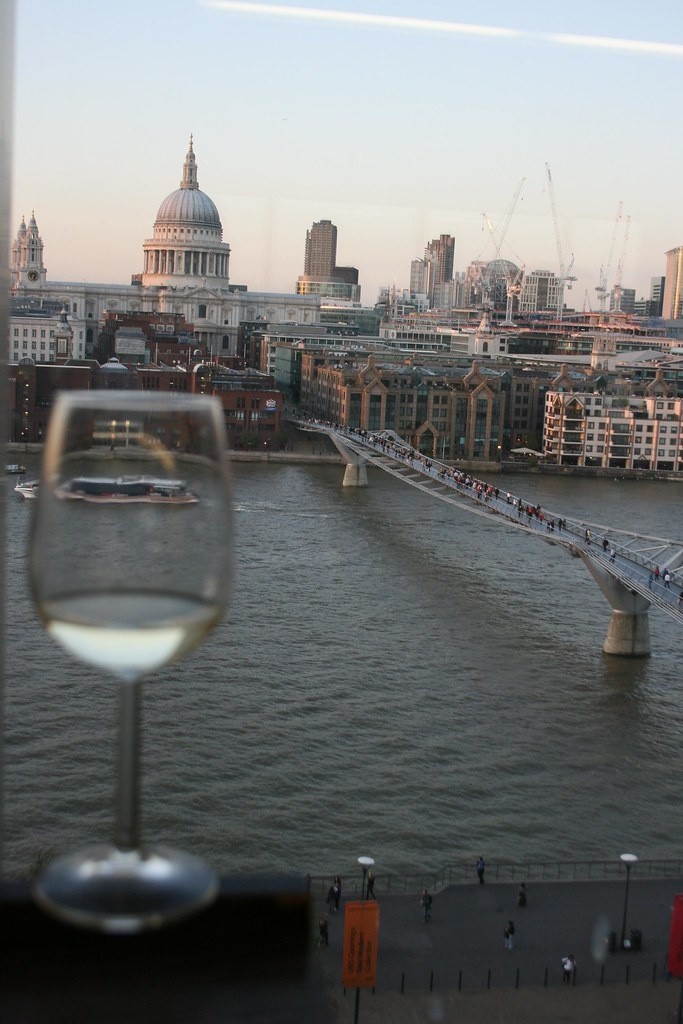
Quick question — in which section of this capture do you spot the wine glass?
[28,386,227,935]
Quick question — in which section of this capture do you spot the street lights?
[355,856,376,1024]
[618,853,639,953]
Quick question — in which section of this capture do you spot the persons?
[504,920,515,949]
[304,417,367,443]
[366,869,377,900]
[437,465,500,503]
[585,528,592,546]
[648,565,670,589]
[547,517,567,533]
[328,876,341,913]
[560,955,576,984]
[318,912,329,945]
[420,457,432,473]
[517,497,544,526]
[477,856,485,885]
[602,537,609,552]
[369,435,414,465]
[420,890,432,922]
[609,548,616,563]
[517,882,527,906]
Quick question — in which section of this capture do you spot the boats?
[4,464,26,474]
[52,474,202,506]
[13,478,41,500]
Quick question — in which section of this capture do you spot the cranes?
[467,155,634,329]
